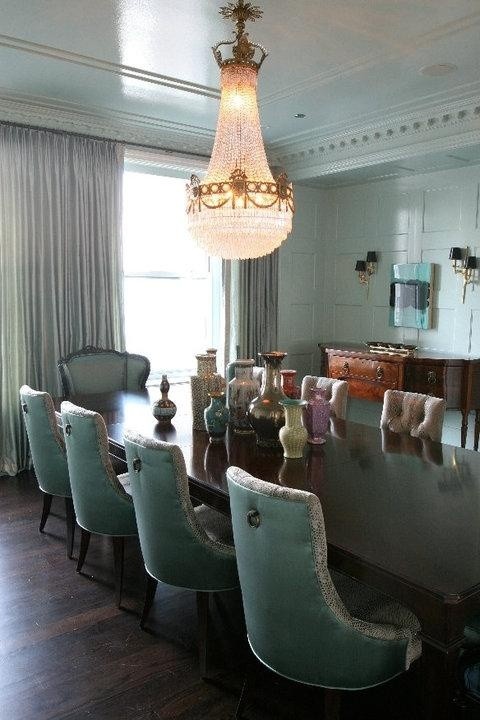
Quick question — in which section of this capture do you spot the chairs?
[60,399,147,607]
[123,427,249,678]
[55,345,150,395]
[251,366,349,421]
[19,384,79,558]
[378,388,446,445]
[222,466,423,718]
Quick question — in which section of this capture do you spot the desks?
[49,380,479,720]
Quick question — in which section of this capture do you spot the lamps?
[183,1,297,263]
[448,246,477,303]
[354,250,380,291]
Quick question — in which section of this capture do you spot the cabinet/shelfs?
[316,341,480,452]
